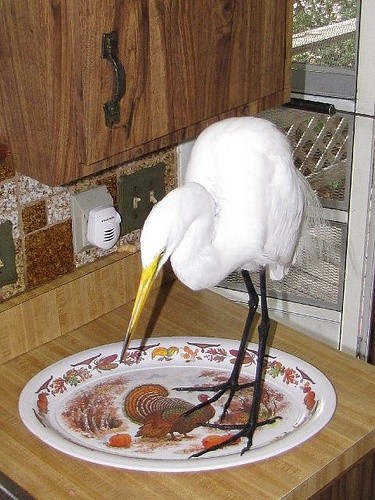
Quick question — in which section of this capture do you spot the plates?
[15,334,337,474]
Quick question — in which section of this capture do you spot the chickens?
[125,384,215,441]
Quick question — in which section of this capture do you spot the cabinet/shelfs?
[0,0,293,193]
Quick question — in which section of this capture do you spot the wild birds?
[119,115,332,461]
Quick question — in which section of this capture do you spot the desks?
[0,244,375,500]
